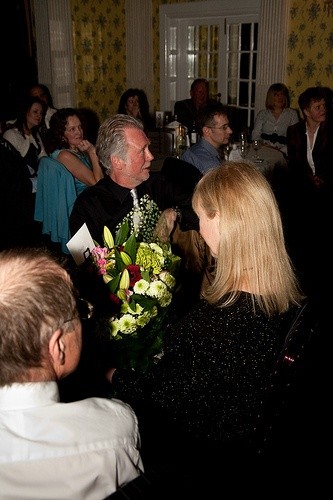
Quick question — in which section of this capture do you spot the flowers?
[91,193,181,341]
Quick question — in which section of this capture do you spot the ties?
[129,188,142,234]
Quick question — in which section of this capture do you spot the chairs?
[159,158,203,220]
[0,137,34,216]
[33,157,79,255]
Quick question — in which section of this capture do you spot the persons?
[100,162,303,456]
[0,246,146,500]
[0,77,333,403]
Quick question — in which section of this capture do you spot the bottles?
[225,146,229,162]
[190,121,197,146]
[229,145,239,161]
[184,127,190,147]
[176,124,185,154]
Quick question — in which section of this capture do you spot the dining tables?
[140,130,290,206]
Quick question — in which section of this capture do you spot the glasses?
[63,296,94,324]
[207,124,229,130]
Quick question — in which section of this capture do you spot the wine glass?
[252,140,260,159]
